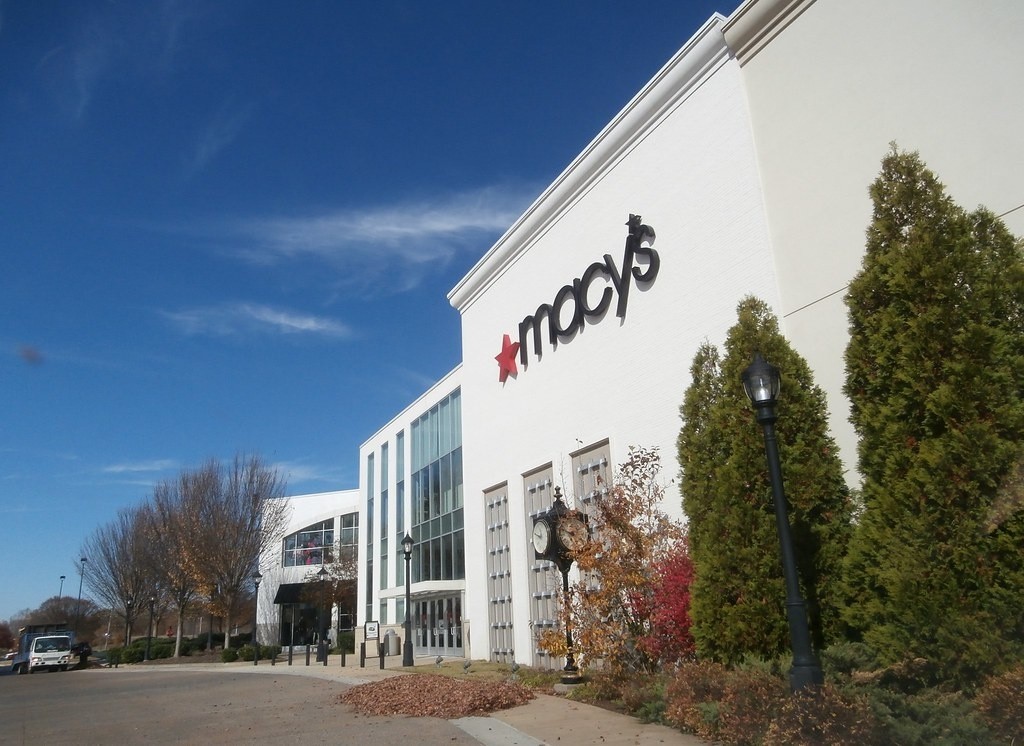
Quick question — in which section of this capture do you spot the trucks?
[11,622,72,674]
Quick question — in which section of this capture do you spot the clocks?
[532,519,550,554]
[556,518,590,552]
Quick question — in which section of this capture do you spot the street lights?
[74,557,88,633]
[316,566,329,662]
[55,575,66,611]
[143,592,157,662]
[123,599,133,646]
[251,570,263,649]
[401,531,417,667]
[737,350,828,694]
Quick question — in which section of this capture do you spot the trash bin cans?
[384,629,401,656]
